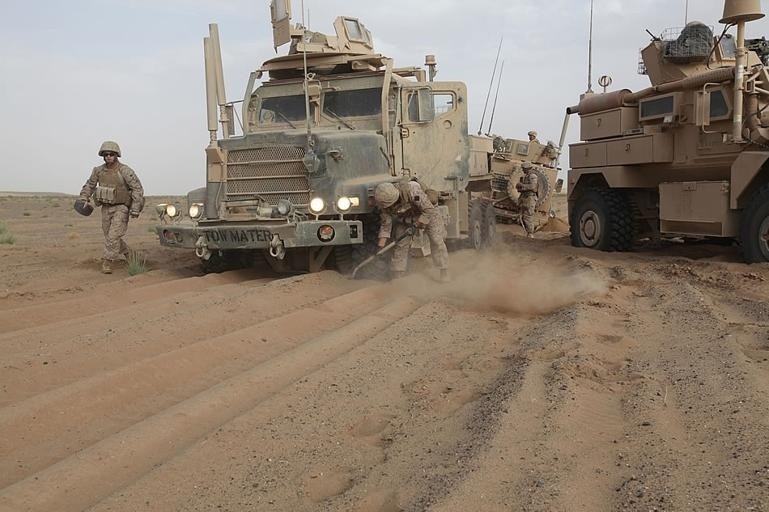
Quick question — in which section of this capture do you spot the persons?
[74,140,145,273]
[516,162,539,238]
[374,180,451,283]
[528,131,540,143]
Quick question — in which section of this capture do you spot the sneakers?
[101,260,112,273]
[440,269,448,282]
[527,232,533,238]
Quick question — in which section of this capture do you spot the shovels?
[345,233,408,279]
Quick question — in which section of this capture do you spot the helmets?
[375,182,399,208]
[99,142,121,157]
[521,162,532,168]
[528,131,537,137]
